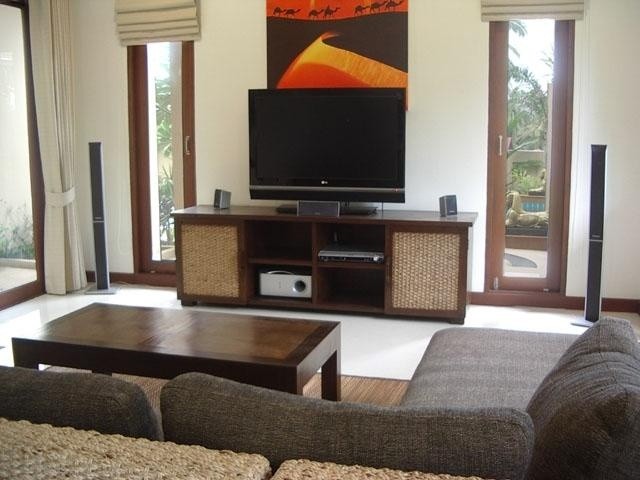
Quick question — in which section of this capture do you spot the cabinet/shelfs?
[170,204,479,323]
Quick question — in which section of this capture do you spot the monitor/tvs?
[247,88,406,215]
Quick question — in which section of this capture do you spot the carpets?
[41,363,410,413]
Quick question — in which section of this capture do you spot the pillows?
[157,371,536,478]
[0,364,164,443]
[526,318,639,479]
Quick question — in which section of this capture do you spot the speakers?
[213,189,232,208]
[83,141,121,295]
[439,195,458,217]
[296,200,341,216]
[571,144,608,330]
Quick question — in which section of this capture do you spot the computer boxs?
[258,272,312,299]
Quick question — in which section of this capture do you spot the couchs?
[0,318,640,479]
